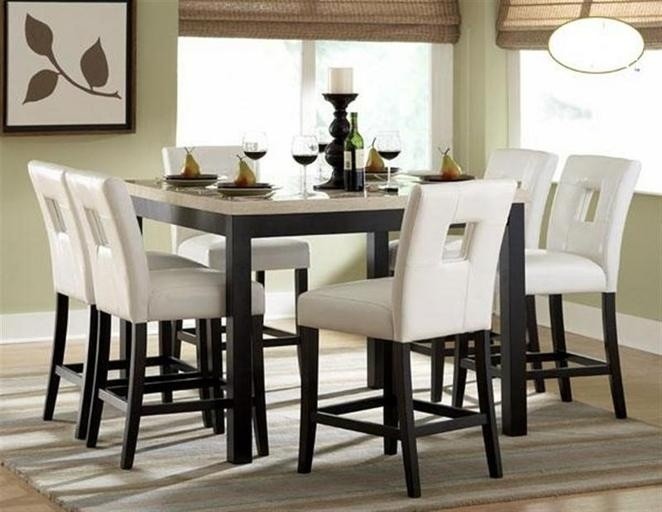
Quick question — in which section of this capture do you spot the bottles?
[338,111,364,191]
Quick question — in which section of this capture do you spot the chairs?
[27,145,642,500]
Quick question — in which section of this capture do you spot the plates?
[418,176,476,182]
[212,182,282,195]
[362,166,399,178]
[161,174,217,187]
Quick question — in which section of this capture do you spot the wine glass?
[308,131,331,182]
[290,141,320,195]
[374,141,401,190]
[244,140,268,187]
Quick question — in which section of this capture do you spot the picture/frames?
[0,0,139,136]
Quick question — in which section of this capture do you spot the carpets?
[0,346,661,512]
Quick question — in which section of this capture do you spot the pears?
[183,146,200,175]
[365,137,384,171]
[438,148,461,179]
[234,154,256,186]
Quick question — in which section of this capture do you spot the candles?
[328,64,355,94]
